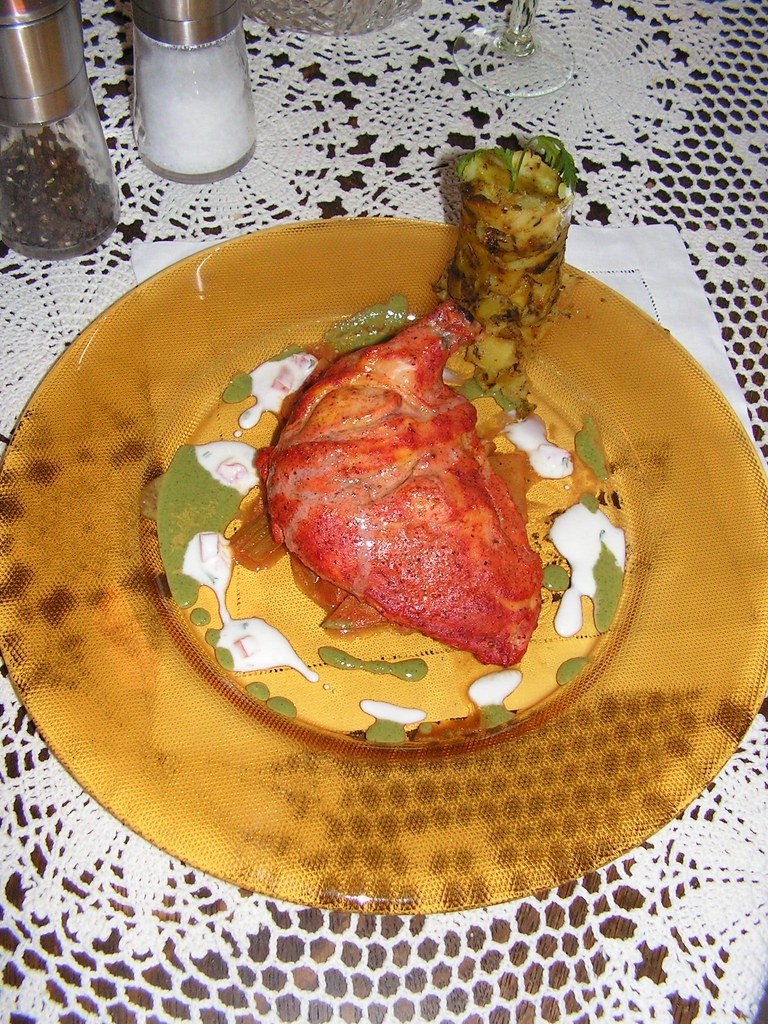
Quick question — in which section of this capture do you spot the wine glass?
[453,0,573,96]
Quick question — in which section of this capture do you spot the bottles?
[0,0,108,258]
[129,0,257,186]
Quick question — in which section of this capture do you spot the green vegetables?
[458,136,579,195]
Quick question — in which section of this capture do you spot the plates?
[0,217,768,913]
